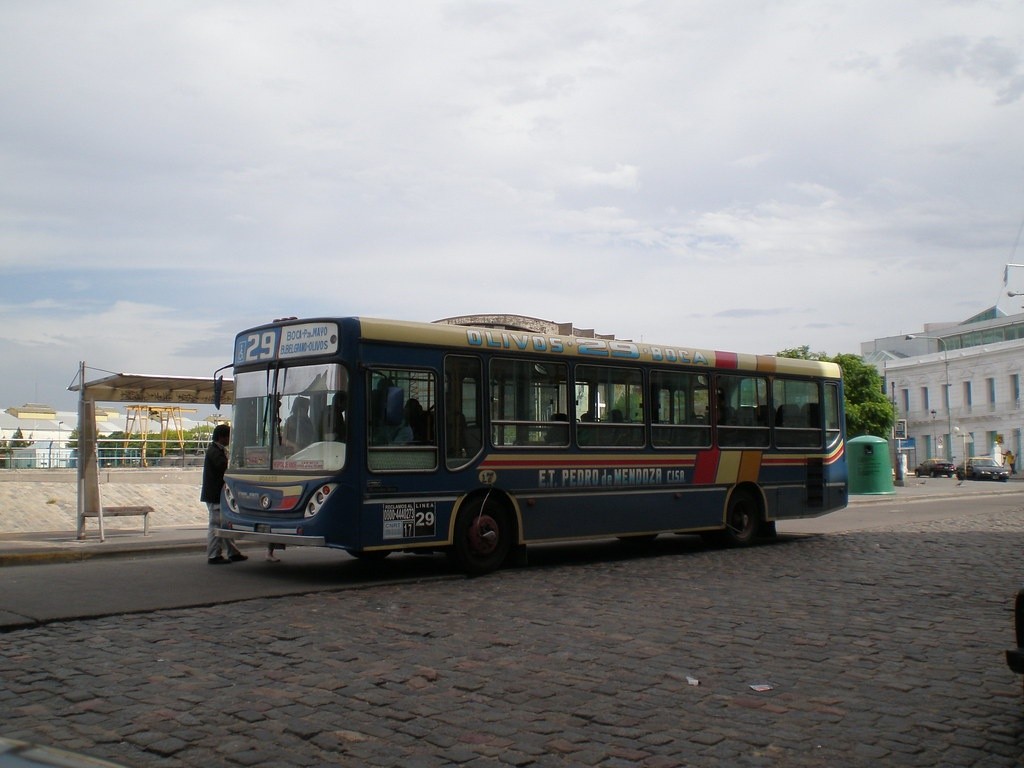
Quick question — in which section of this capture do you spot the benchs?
[82,506,154,539]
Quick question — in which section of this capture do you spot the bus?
[211,311,849,581]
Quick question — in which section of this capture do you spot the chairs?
[726,404,819,446]
[548,409,708,446]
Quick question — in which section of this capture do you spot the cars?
[956,457,1009,483]
[915,459,954,478]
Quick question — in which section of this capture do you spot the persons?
[280,396,313,449]
[1006,589,1024,674]
[319,390,347,442]
[371,378,430,443]
[544,410,623,446]
[717,392,727,426]
[1001,451,1017,476]
[267,543,286,562]
[201,425,249,565]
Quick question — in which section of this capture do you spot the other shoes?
[229,554,249,561]
[208,555,231,564]
[266,555,280,561]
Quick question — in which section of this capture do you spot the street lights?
[48,441,53,468]
[891,381,898,438]
[58,421,64,468]
[954,424,967,480]
[905,332,954,479]
